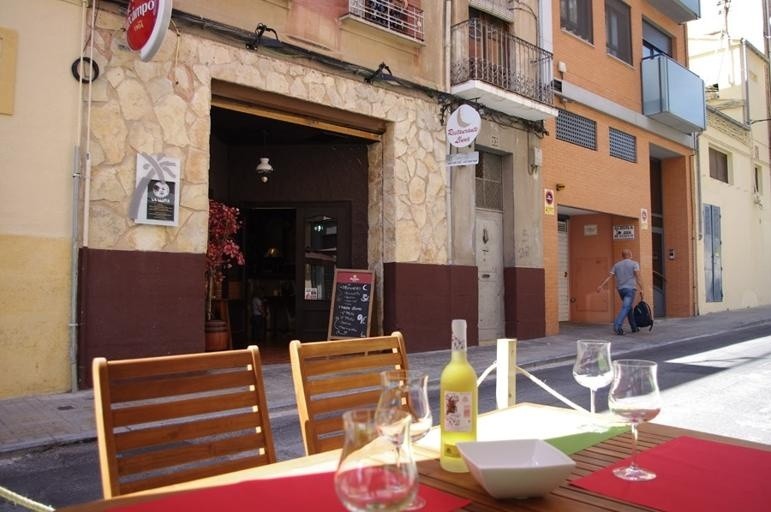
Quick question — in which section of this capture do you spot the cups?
[609,360,661,481]
[570,340,614,433]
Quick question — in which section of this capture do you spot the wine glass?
[375,371,432,509]
[334,410,418,512]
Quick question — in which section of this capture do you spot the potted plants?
[207,199,245,352]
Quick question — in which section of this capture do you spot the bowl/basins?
[459,440,575,498]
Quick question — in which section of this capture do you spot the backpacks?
[634,301,653,331]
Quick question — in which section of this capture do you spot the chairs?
[288,331,422,457]
[93,345,277,500]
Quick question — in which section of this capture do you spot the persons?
[251,287,266,343]
[596,248,644,335]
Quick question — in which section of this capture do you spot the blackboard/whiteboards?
[327,269,376,340]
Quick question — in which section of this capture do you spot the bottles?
[439,320,477,475]
